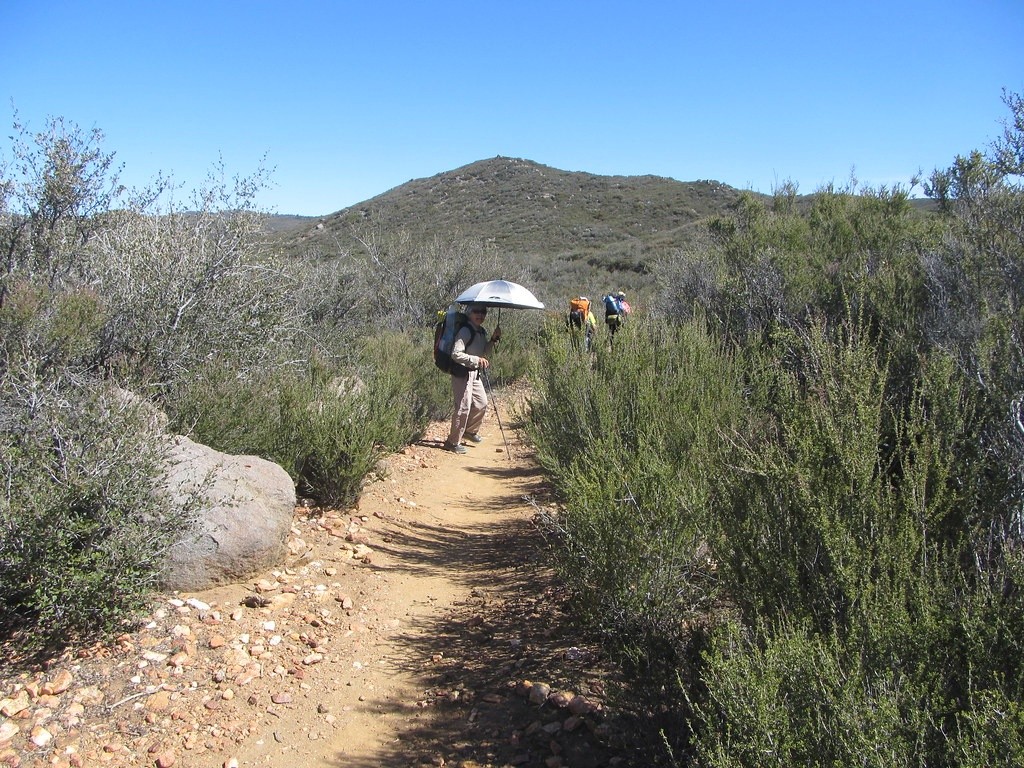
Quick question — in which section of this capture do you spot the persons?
[444,302,501,454]
[569,297,597,353]
[601,291,631,351]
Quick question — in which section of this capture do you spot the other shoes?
[443,439,467,454]
[462,431,482,443]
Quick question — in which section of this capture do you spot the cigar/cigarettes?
[491,337,497,342]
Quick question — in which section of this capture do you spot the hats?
[618,291,626,297]
[579,297,592,308]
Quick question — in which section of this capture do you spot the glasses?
[471,309,488,314]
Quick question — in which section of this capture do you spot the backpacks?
[433,311,481,380]
[616,299,624,312]
[604,295,620,315]
[569,299,589,326]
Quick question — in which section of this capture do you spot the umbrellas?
[454,278,545,343]
